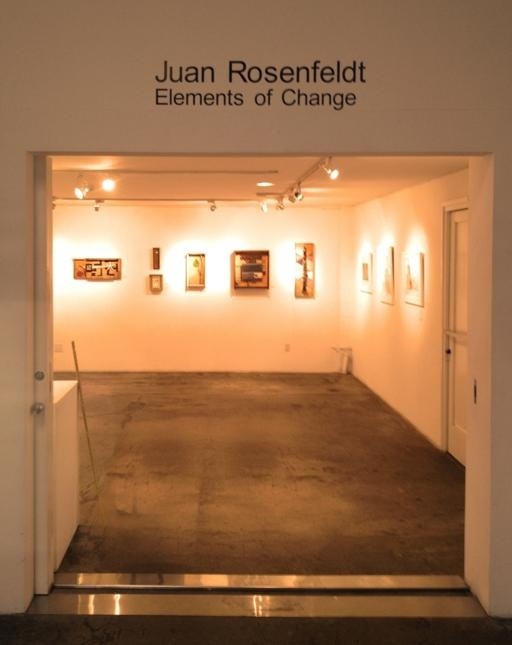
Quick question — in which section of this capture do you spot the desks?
[53,380,78,573]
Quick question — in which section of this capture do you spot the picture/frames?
[70,241,423,306]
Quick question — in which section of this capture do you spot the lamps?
[74,158,339,212]
